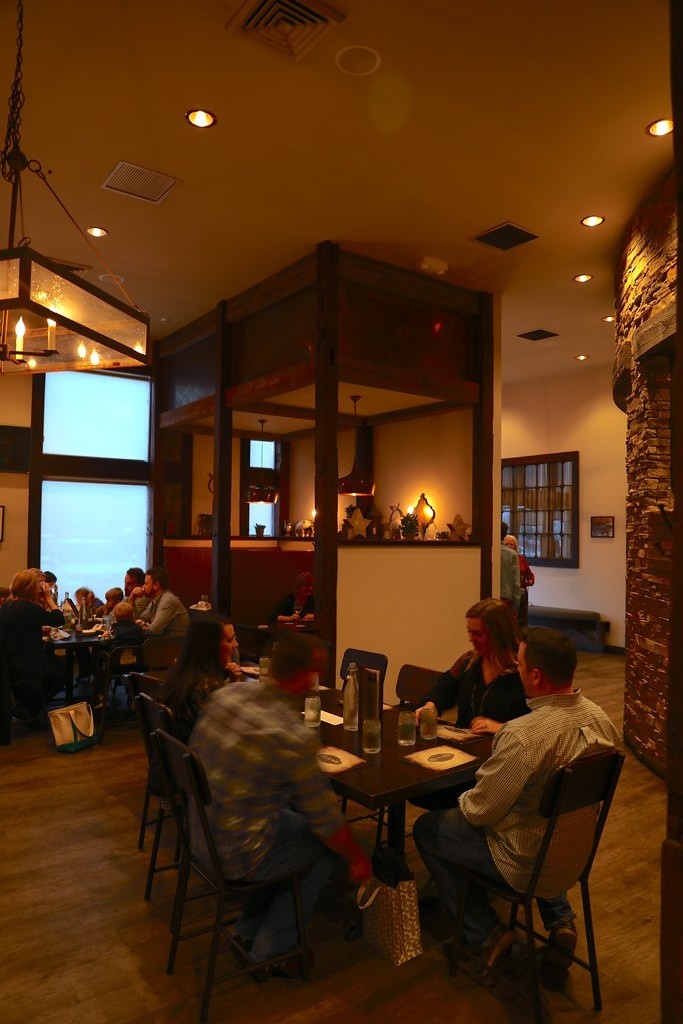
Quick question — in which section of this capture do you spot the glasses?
[302,584,314,588]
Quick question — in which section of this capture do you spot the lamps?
[0,0,151,375]
[243,419,278,503]
[337,395,375,496]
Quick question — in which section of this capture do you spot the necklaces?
[294,601,303,615]
[470,658,517,718]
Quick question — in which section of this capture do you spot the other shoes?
[476,927,514,976]
[545,921,576,969]
[248,954,311,983]
[231,935,253,958]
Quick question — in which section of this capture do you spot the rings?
[484,724,487,728]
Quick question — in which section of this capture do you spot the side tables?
[257,620,315,662]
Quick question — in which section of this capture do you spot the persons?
[94,601,145,711]
[0,586,11,609]
[74,588,105,682]
[120,567,191,722]
[108,568,152,622]
[413,627,622,992]
[408,596,529,812]
[29,567,49,609]
[268,571,318,625]
[500,522,520,611]
[503,534,535,592]
[85,588,123,615]
[0,570,66,730]
[158,612,243,817]
[188,647,374,985]
[43,572,58,605]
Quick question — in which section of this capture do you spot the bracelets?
[137,620,142,626]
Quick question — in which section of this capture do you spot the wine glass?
[294,601,303,624]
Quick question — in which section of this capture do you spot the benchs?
[527,604,610,654]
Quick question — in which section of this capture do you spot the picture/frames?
[591,516,614,537]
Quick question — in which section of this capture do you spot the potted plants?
[399,512,418,539]
[255,523,265,536]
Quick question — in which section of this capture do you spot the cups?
[420,706,438,740]
[304,696,321,727]
[397,710,416,747]
[362,717,381,754]
[102,618,111,632]
[76,624,83,638]
[259,656,271,683]
[42,626,51,640]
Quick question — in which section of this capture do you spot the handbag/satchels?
[357,875,421,965]
[48,701,100,752]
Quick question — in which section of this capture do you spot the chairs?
[448,749,626,1024]
[340,663,447,856]
[0,633,187,747]
[150,727,311,1023]
[135,692,183,899]
[340,648,388,702]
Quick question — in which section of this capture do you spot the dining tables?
[42,628,117,724]
[135,662,495,874]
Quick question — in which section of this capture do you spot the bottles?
[343,663,360,731]
[87,607,94,630]
[63,592,72,632]
[78,597,89,630]
